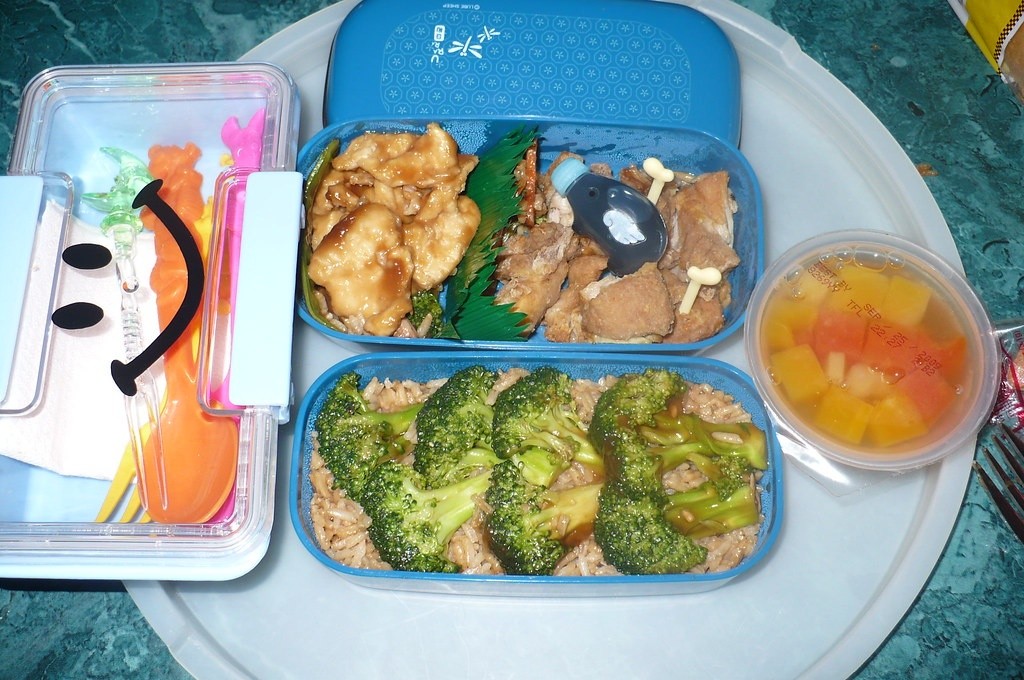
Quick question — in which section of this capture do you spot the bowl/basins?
[288,354,784,599]
[0,62,311,584]
[744,231,1002,471]
[295,115,767,354]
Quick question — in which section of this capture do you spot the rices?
[309,374,762,575]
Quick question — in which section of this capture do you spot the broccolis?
[316,376,767,577]
